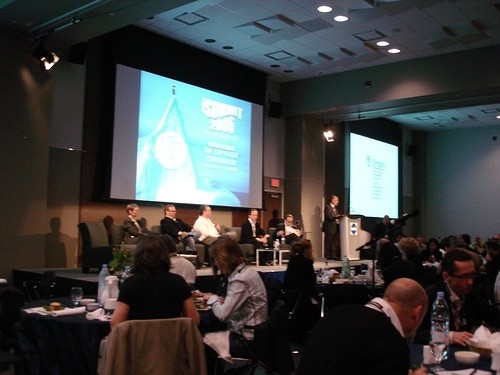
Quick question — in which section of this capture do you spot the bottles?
[321,256,376,283]
[281,235,285,245]
[430,292,450,360]
[275,239,280,250]
[97,264,110,302]
[121,265,133,283]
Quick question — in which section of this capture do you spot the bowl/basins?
[79,299,95,306]
[469,345,492,358]
[86,303,99,311]
[454,351,480,363]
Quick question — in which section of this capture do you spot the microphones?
[390,213,410,224]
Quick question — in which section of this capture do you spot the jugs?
[101,275,119,307]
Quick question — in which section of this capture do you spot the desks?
[12,296,114,375]
[256,248,291,266]
[423,342,497,375]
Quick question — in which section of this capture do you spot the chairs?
[104,317,206,375]
[77,222,123,274]
[153,225,204,262]
[269,227,292,259]
[231,226,253,251]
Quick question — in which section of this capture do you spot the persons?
[376,233,500,304]
[238,209,273,266]
[194,235,268,375]
[108,237,200,332]
[375,215,397,243]
[322,195,343,262]
[193,206,237,245]
[294,277,428,375]
[122,203,149,243]
[160,205,207,268]
[272,214,303,244]
[282,239,317,342]
[414,249,478,346]
[158,234,196,286]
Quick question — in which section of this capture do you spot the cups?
[423,344,434,364]
[104,299,117,310]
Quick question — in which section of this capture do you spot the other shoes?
[199,260,208,265]
[261,261,271,266]
[327,255,340,261]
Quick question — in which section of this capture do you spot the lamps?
[41,52,59,70]
[322,120,335,142]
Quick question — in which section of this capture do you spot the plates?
[434,368,492,375]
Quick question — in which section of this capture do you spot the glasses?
[447,271,478,280]
[167,209,176,213]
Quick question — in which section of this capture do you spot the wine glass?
[430,341,448,372]
[71,287,82,308]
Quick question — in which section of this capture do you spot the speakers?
[269,101,282,118]
[69,41,88,64]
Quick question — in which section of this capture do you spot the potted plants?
[109,241,135,276]
